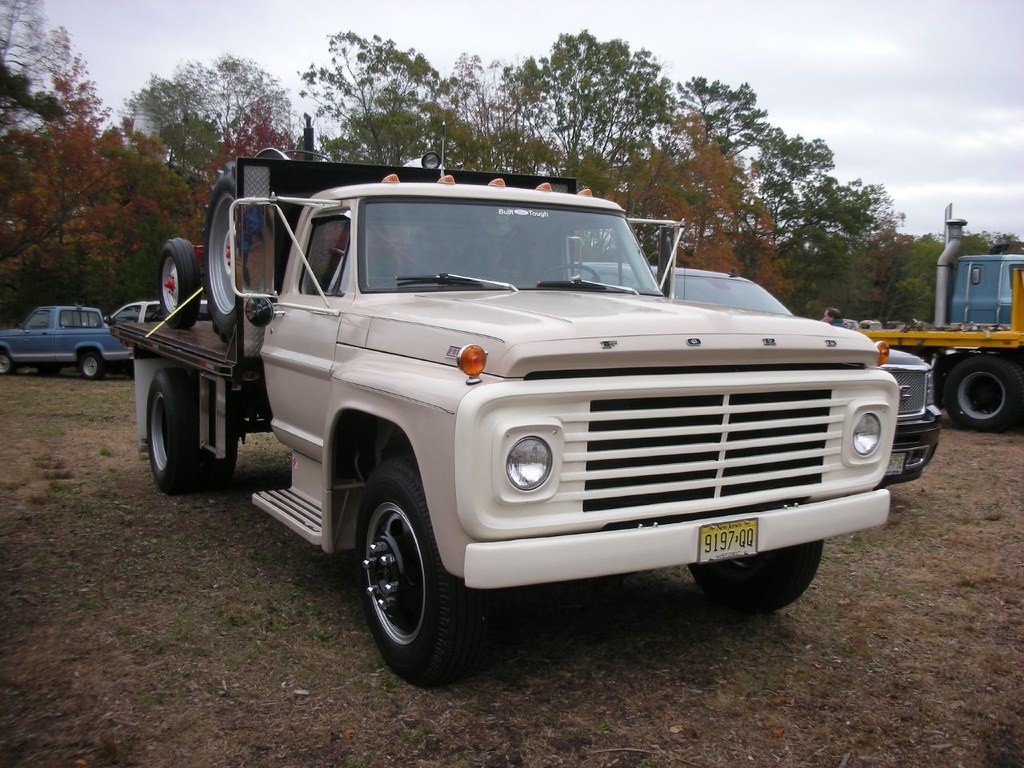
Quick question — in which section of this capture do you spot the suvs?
[573,262,942,491]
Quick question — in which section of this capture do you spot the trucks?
[107,155,902,690]
[853,218,1024,433]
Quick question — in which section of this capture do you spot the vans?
[946,241,1024,324]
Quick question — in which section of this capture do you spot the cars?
[0,304,134,381]
[82,299,208,378]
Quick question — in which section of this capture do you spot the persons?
[823,308,849,329]
